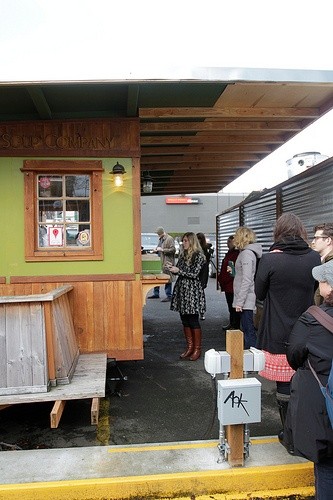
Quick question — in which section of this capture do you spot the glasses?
[314,236,329,240]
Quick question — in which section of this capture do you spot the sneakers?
[161,297,172,302]
[148,295,159,298]
[199,314,205,320]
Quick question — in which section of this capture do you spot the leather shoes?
[222,324,239,330]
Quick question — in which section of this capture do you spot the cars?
[174,237,216,277]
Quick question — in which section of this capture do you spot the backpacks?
[305,305,333,430]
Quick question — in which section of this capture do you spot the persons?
[227,227,262,350]
[255,212,323,454]
[199,232,213,319]
[148,226,176,302]
[284,259,333,500]
[218,236,241,330]
[311,223,333,306]
[165,232,202,361]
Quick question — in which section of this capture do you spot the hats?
[155,226,164,232]
[312,259,333,289]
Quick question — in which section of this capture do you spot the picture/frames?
[48,227,63,246]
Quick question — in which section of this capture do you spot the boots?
[180,327,201,361]
[276,392,290,445]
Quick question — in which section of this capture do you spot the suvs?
[141,233,160,255]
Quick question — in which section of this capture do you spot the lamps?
[109,161,127,187]
